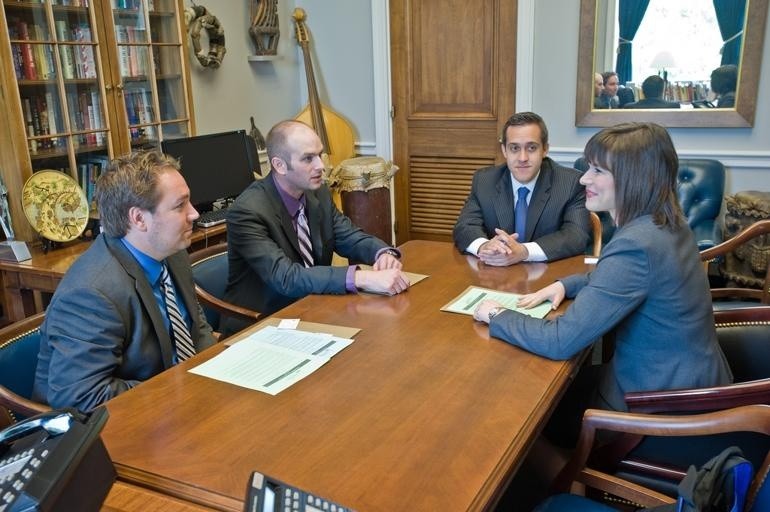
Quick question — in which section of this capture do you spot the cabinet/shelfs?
[0,1,199,243]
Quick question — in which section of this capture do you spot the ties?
[610,97,617,108]
[297,203,315,268]
[515,187,530,243]
[160,264,197,364]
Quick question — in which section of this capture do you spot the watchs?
[377,250,398,259]
[488,306,508,323]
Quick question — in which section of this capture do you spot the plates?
[21,168,89,242]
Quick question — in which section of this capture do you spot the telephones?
[243,469,363,511]
[0,403,121,512]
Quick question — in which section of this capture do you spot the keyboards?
[193,207,228,228]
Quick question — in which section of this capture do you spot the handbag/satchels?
[633,444,754,511]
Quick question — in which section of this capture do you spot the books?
[627,80,711,101]
[9,0,171,141]
[49,155,147,212]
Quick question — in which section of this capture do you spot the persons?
[600,71,620,107]
[31,152,220,419]
[624,74,679,109]
[448,112,592,267]
[472,124,734,500]
[709,64,738,109]
[215,119,410,345]
[592,71,607,109]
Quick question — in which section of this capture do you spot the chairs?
[532,404,770,509]
[588,211,602,258]
[699,220,770,305]
[573,159,724,275]
[601,306,770,500]
[0,309,224,429]
[188,243,264,332]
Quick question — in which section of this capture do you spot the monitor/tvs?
[160,129,255,210]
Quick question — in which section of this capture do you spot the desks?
[90,238,598,511]
[0,211,230,326]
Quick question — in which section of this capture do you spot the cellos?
[293,8,356,214]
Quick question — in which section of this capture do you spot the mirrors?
[574,1,769,130]
